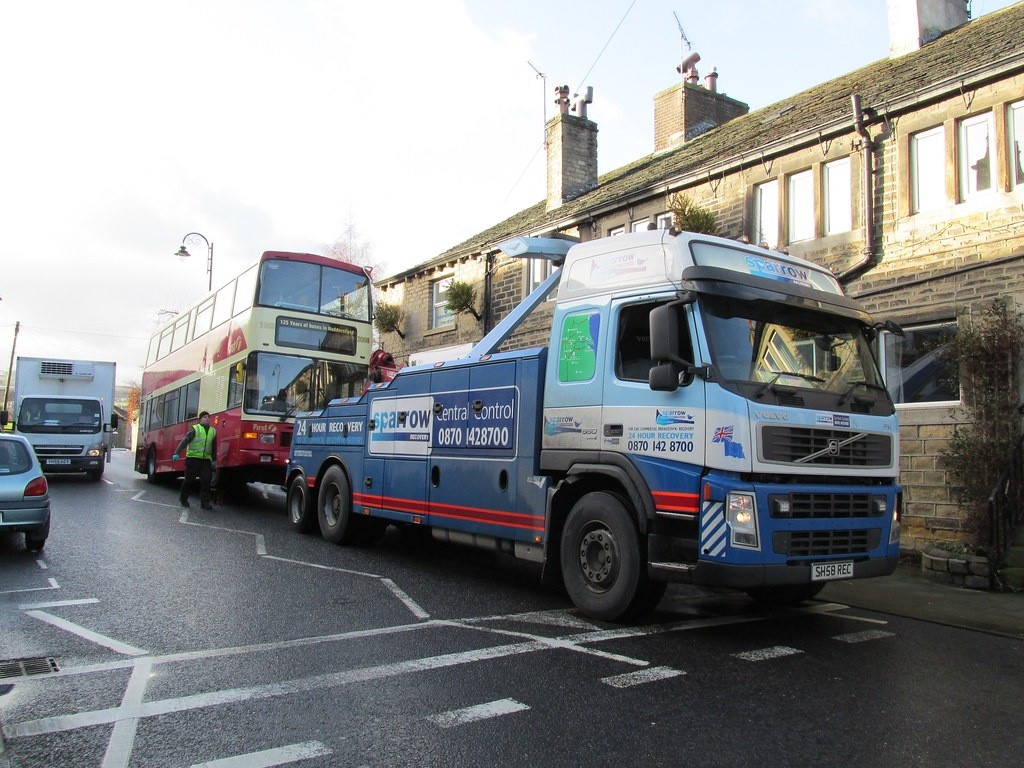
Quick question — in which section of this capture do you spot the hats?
[198,411,211,419]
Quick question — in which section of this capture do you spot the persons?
[25,402,44,422]
[171,411,217,511]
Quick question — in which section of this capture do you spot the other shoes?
[179,497,190,508]
[201,501,213,510]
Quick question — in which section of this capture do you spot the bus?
[134,250,374,506]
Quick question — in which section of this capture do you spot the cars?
[0,433,50,550]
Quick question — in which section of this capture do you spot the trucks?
[14,356,117,481]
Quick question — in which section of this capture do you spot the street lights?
[174,232,213,294]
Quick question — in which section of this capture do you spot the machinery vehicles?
[283,227,906,623]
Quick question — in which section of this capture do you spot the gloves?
[212,461,216,469]
[172,454,179,463]
